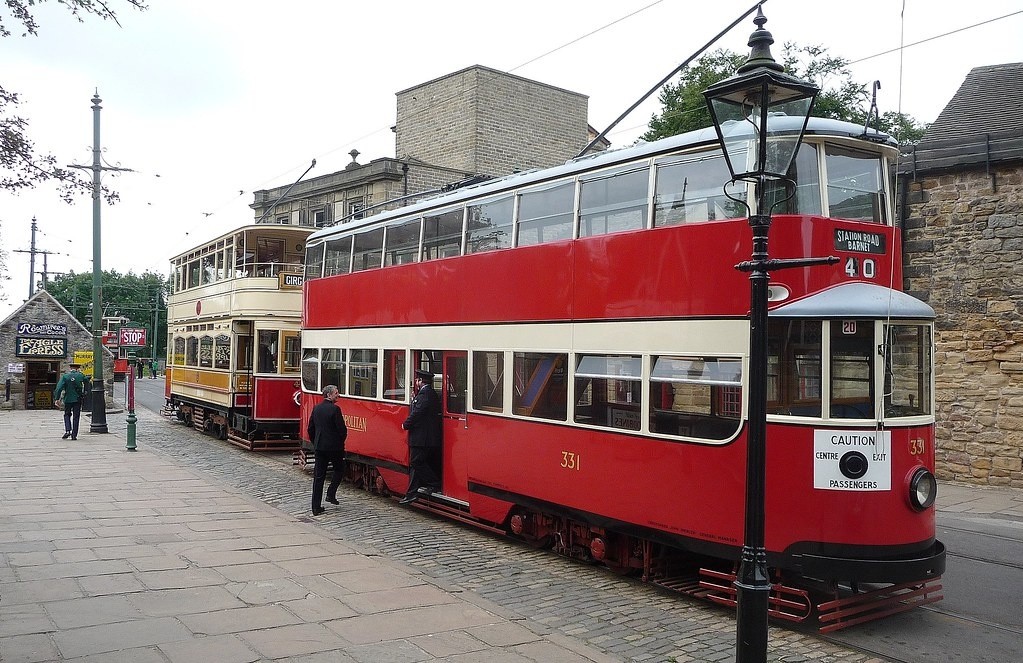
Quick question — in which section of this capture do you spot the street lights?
[696,6,824,663]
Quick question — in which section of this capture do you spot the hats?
[69,363,81,367]
[415,369,435,380]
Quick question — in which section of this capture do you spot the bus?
[158,159,322,452]
[96,317,130,381]
[299,1,949,635]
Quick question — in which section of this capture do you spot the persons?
[258,337,278,373]
[309,384,348,517]
[137,357,159,380]
[398,369,440,504]
[53,364,93,440]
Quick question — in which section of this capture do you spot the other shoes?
[311,506,324,515]
[62,432,70,438]
[399,492,419,505]
[72,436,78,440]
[326,496,340,505]
